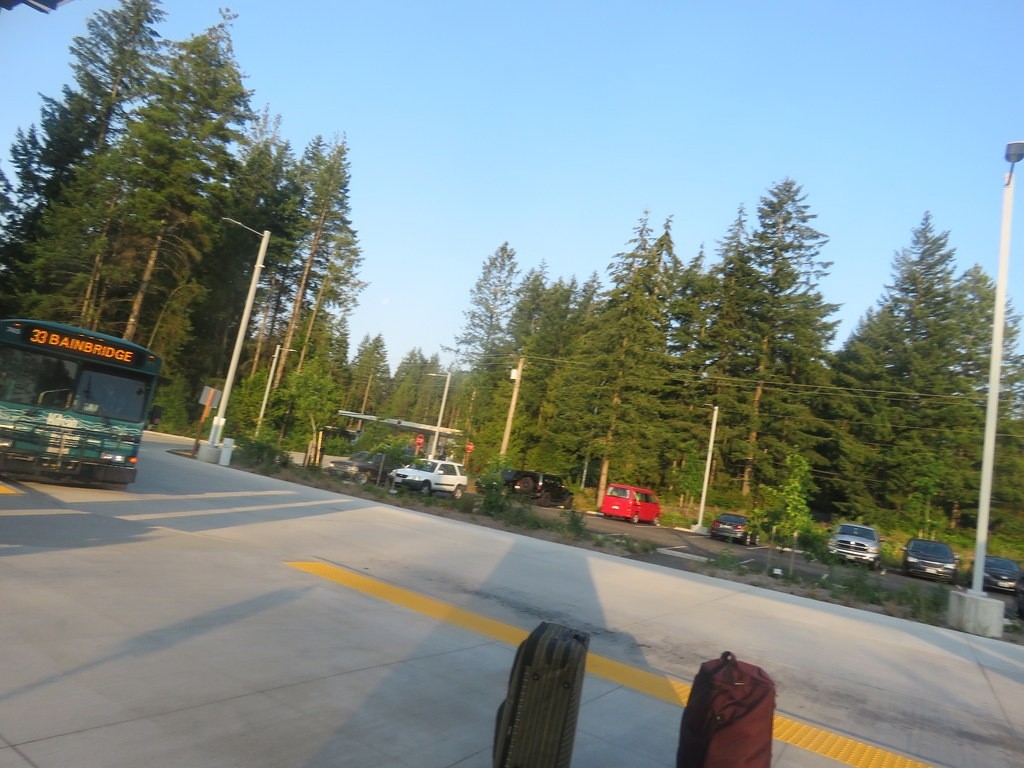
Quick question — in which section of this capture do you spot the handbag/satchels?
[675,652,775,768]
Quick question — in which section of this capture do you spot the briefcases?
[493,622,593,768]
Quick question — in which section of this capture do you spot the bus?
[0,318,163,483]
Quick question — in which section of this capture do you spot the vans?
[600,483,661,527]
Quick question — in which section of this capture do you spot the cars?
[330,450,401,486]
[712,513,760,547]
[902,538,962,584]
[968,556,1023,595]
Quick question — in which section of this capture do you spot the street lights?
[949,140,1024,640]
[253,344,297,438]
[427,371,453,459]
[196,216,271,465]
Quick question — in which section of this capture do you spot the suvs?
[387,459,468,499]
[827,523,886,571]
[502,471,574,510]
